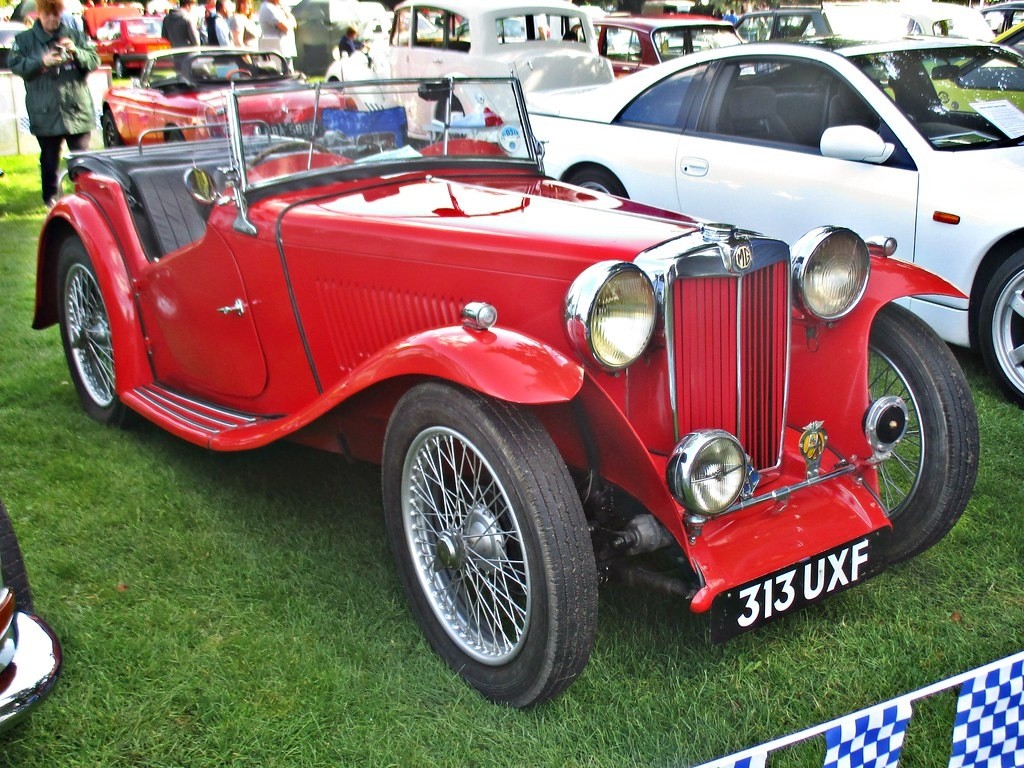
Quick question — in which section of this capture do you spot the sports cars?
[103,45,360,148]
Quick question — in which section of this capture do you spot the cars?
[324,0,1024,167]
[466,30,1024,406]
[29,72,980,713]
[0,21,28,74]
[81,5,177,80]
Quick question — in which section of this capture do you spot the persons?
[8,0,101,207]
[11,0,450,78]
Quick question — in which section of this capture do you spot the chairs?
[826,79,895,149]
[729,84,797,150]
[933,64,965,89]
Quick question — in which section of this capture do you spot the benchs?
[127,152,325,253]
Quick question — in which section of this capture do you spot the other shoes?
[44,199,56,212]
